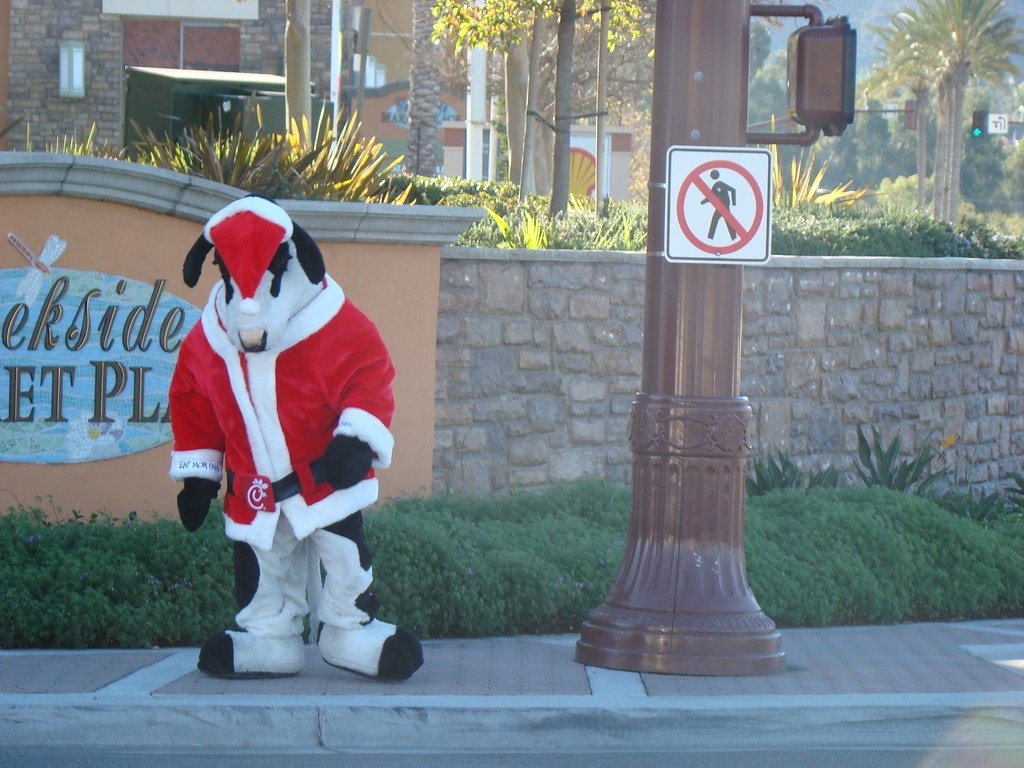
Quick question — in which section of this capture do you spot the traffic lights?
[968,109,987,138]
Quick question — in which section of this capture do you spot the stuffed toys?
[170,194,424,681]
